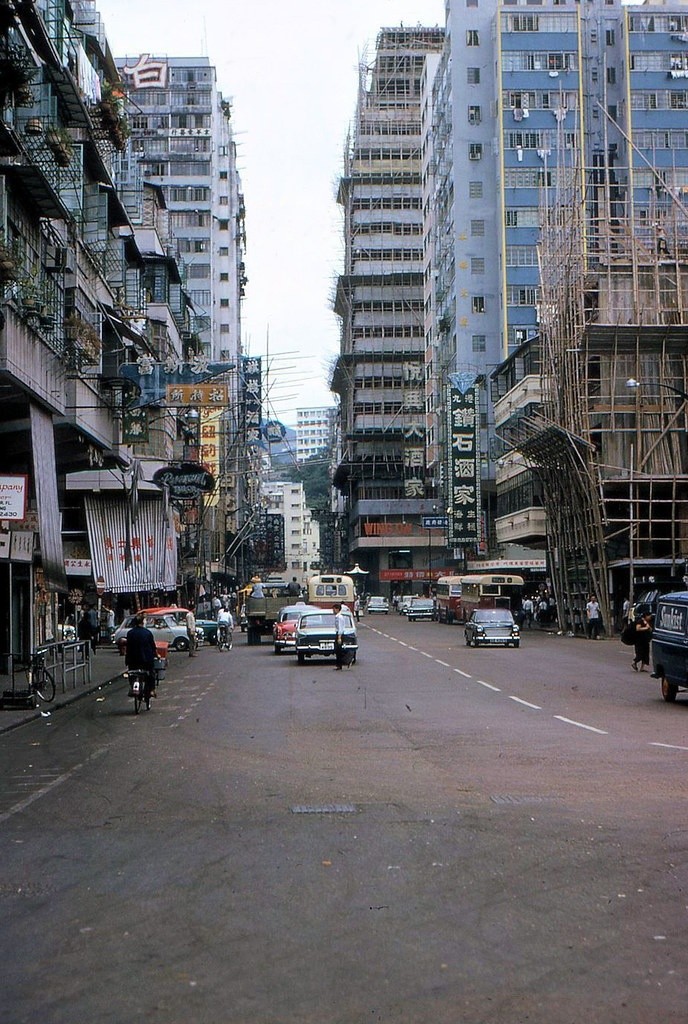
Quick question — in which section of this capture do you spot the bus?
[436,576,462,624]
[459,574,524,628]
[306,575,355,616]
[236,585,255,621]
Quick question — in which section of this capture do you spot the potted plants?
[0,0,135,363]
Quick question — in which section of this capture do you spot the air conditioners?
[469,152,480,159]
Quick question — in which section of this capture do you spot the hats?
[250,576,261,583]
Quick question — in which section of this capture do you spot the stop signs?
[97,576,106,595]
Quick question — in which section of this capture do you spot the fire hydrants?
[118,638,126,656]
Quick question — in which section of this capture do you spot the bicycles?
[129,671,155,714]
[28,651,56,709]
[217,625,234,652]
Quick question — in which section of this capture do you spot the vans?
[650,589,688,701]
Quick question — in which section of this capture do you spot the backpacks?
[620,618,641,646]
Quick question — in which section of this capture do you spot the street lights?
[403,521,432,594]
[224,504,258,610]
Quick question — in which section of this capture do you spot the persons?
[586,595,601,640]
[63,592,242,698]
[287,577,301,597]
[333,603,354,671]
[623,597,652,673]
[341,592,436,623]
[522,587,560,629]
[249,575,265,599]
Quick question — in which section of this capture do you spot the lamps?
[149,407,200,426]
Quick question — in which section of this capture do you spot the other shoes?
[631,663,638,672]
[640,669,648,672]
[333,667,342,670]
[348,658,354,669]
[189,652,198,657]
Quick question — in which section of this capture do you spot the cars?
[368,597,390,615]
[240,605,249,631]
[272,605,323,653]
[397,596,438,622]
[111,606,219,651]
[294,610,360,663]
[464,609,521,648]
[58,625,76,645]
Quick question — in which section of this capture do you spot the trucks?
[246,584,305,645]
